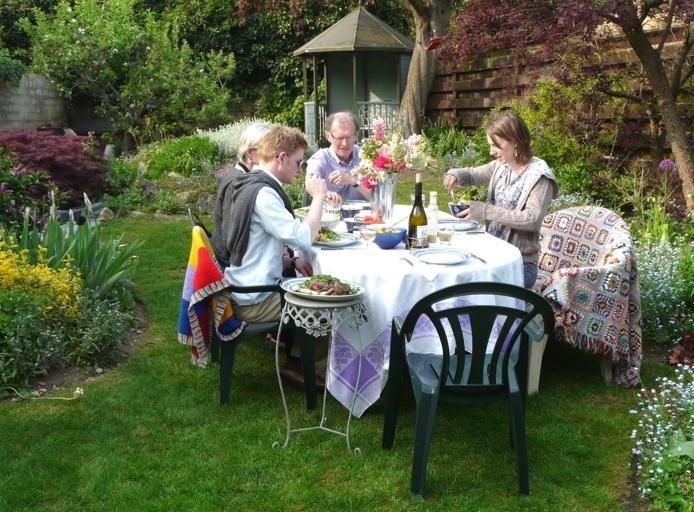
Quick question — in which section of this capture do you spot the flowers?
[351,117,432,189]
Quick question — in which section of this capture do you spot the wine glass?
[360,225,376,256]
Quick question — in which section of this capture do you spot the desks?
[272,293,367,456]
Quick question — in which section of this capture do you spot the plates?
[279,276,364,302]
[408,247,469,267]
[292,198,378,224]
[438,219,478,232]
[314,233,360,248]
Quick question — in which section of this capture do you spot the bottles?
[409,194,416,211]
[420,193,426,209]
[408,171,429,249]
[427,190,439,237]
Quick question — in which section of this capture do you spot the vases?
[372,173,399,221]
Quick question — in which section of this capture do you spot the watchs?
[353,180,361,187]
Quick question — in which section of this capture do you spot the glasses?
[276,152,304,168]
[331,133,356,141]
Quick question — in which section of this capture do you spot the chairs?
[382,282,554,497]
[188,207,316,413]
[477,205,641,395]
[302,176,396,211]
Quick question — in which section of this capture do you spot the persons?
[305,112,374,204]
[443,114,559,289]
[215,122,326,391]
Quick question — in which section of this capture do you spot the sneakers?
[265,333,287,350]
[280,362,324,393]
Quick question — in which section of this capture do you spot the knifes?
[470,252,485,265]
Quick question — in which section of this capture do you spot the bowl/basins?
[321,213,341,229]
[446,203,470,220]
[373,227,406,250]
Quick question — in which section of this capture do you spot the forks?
[399,254,412,266]
